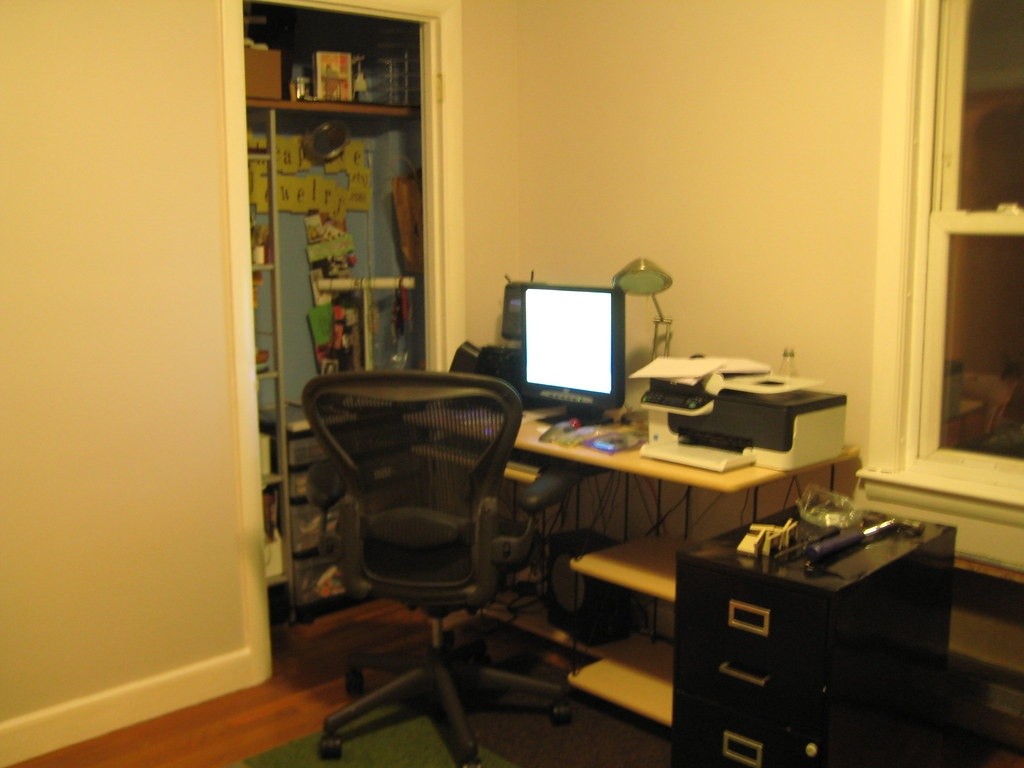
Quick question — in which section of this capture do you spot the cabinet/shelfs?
[568,533,704,722]
[671,498,957,768]
[262,410,347,604]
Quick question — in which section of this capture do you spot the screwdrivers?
[773,525,841,560]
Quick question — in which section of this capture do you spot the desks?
[405,406,858,726]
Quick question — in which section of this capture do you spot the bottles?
[776,348,800,378]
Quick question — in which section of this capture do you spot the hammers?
[805,512,925,559]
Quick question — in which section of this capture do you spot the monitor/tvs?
[520,283,626,431]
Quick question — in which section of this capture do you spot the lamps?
[612,259,674,402]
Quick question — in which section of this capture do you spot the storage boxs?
[243,48,281,100]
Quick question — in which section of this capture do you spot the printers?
[638,371,847,472]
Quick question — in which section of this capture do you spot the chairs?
[300,370,576,768]
